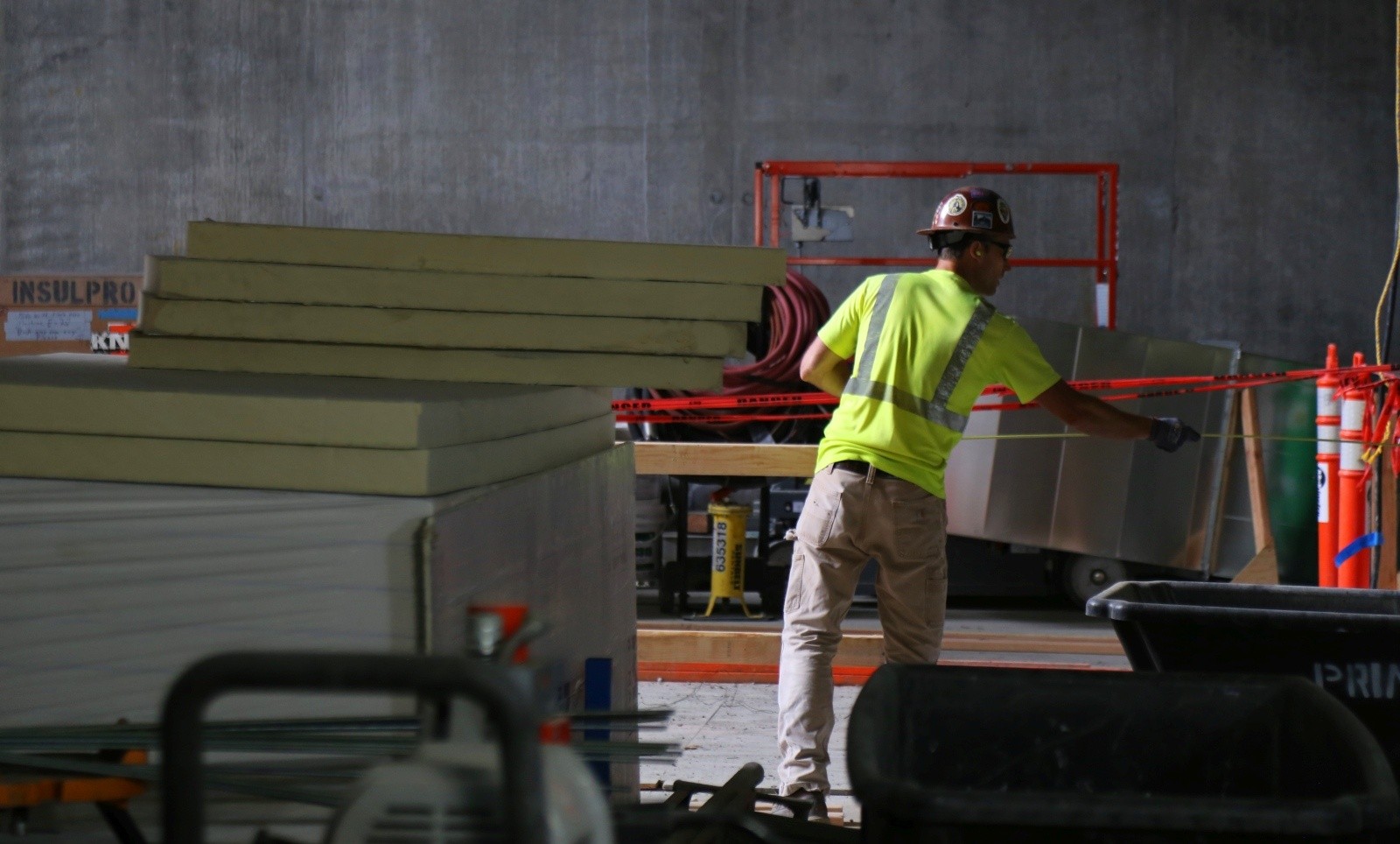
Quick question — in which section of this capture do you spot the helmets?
[916,186,1017,242]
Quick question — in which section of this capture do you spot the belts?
[834,460,898,479]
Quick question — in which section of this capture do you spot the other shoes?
[771,790,830,824]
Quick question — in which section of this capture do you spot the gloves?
[1148,417,1200,453]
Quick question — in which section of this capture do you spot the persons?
[774,186,1204,820]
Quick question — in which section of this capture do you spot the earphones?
[974,249,981,258]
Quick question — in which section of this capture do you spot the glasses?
[981,234,1013,260]
